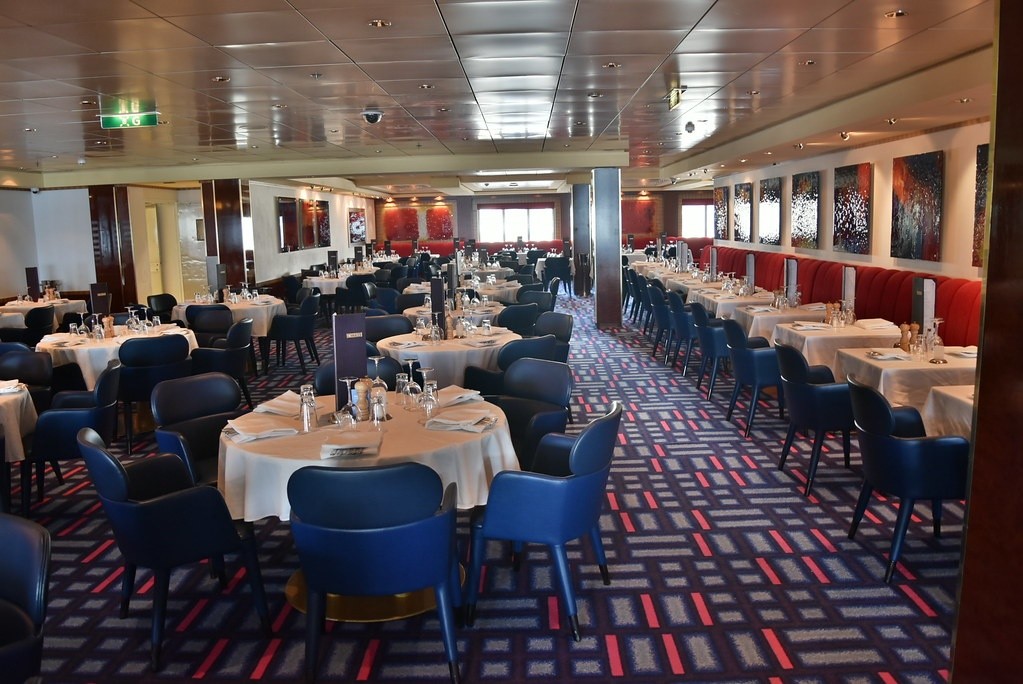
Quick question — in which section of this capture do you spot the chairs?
[0,251,928,684]
[846,372,969,580]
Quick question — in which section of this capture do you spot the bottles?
[413,245,431,256]
[505,243,558,257]
[424,291,488,312]
[925,329,933,352]
[373,248,396,260]
[416,313,477,338]
[347,254,373,271]
[839,299,854,328]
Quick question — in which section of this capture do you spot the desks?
[0,253,977,625]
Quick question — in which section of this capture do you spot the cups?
[423,380,439,403]
[70,324,79,338]
[334,411,352,426]
[831,310,840,328]
[481,319,492,336]
[194,292,200,302]
[473,273,496,289]
[152,316,160,326]
[417,394,436,420]
[318,264,353,280]
[92,324,102,339]
[773,285,800,309]
[909,334,945,360]
[230,293,237,304]
[79,330,87,342]
[246,293,252,300]
[371,396,387,431]
[252,289,260,299]
[395,374,408,405]
[300,384,319,433]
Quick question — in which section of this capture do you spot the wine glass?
[416,366,437,410]
[930,317,944,347]
[339,376,362,422]
[492,256,498,269]
[239,282,251,299]
[125,306,153,333]
[429,312,444,344]
[16,287,33,303]
[402,358,421,410]
[200,284,214,304]
[651,249,752,295]
[227,285,233,303]
[368,356,388,402]
[76,311,103,339]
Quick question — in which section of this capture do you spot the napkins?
[423,409,498,433]
[411,326,431,336]
[476,327,510,335]
[222,419,297,444]
[474,307,496,314]
[697,288,720,295]
[0,380,28,393]
[252,296,276,305]
[753,292,775,299]
[744,305,779,313]
[154,323,188,337]
[853,317,898,331]
[421,385,483,406]
[421,281,431,286]
[416,309,432,315]
[45,298,69,304]
[498,280,517,288]
[674,276,691,281]
[254,390,323,418]
[410,283,426,290]
[5,299,34,306]
[320,432,382,459]
[494,279,508,284]
[463,337,499,347]
[713,293,737,300]
[787,321,833,330]
[384,341,429,350]
[865,351,912,360]
[481,300,501,306]
[944,345,979,357]
[796,302,827,310]
[683,280,702,285]
[50,337,88,348]
[113,335,147,345]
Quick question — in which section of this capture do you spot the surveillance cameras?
[685,125,695,133]
[485,184,489,186]
[31,188,40,194]
[361,112,385,124]
